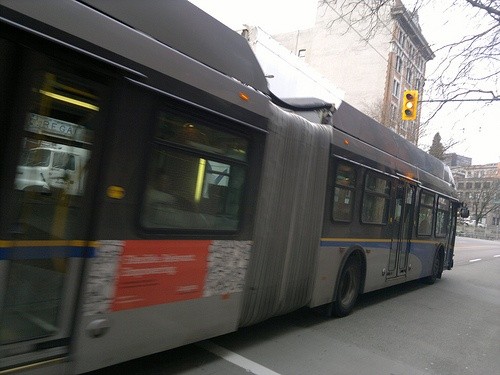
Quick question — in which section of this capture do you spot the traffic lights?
[401,90,418,120]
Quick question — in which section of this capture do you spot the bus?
[0,0,469,375]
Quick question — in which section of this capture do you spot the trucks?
[14,147,86,196]
